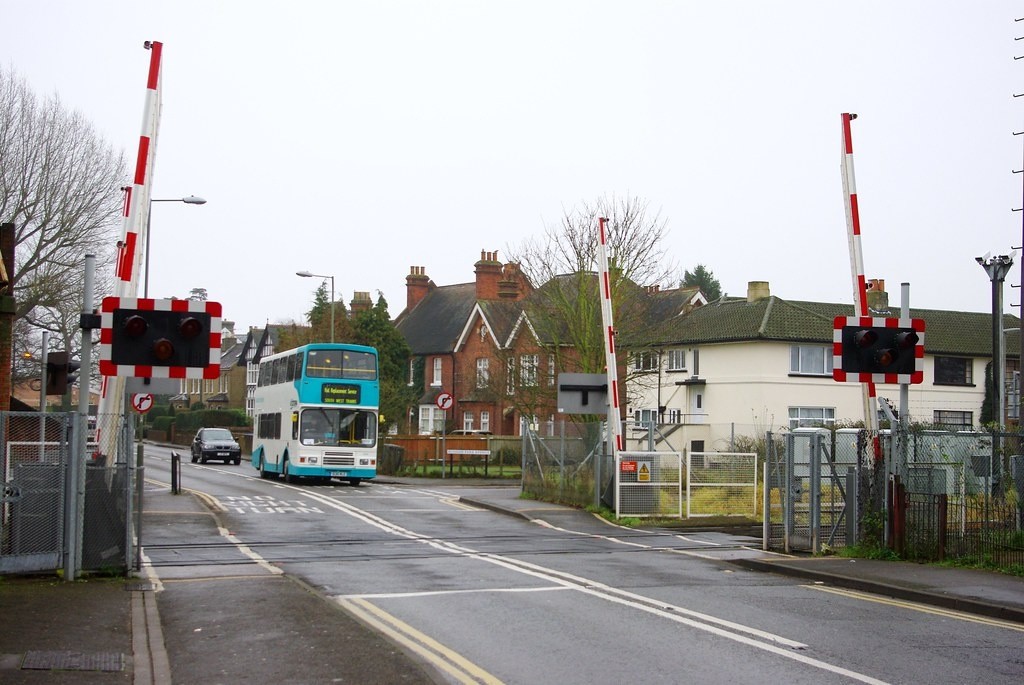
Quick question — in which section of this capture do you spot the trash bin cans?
[383,444,404,474]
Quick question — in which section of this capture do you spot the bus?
[251,344,385,484]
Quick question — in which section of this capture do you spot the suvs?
[191,427,241,465]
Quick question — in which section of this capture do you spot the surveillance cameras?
[1000,249,1017,264]
[975,250,990,264]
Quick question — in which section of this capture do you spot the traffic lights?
[112,308,209,367]
[48,351,82,394]
[842,326,920,374]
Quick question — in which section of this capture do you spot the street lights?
[296,271,334,343]
[135,195,207,572]
